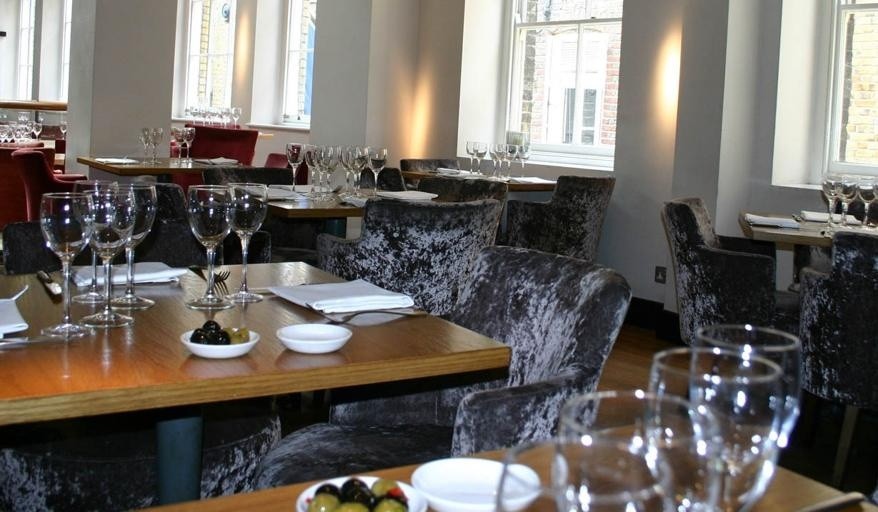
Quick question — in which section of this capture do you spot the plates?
[411,455,541,511]
[295,472,429,511]
[180,328,263,361]
[276,325,354,353]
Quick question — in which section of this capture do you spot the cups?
[694,324,805,449]
[559,390,722,511]
[502,433,676,512]
[648,348,783,512]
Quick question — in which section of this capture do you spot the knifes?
[32,268,64,298]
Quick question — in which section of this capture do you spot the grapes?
[308,477,408,512]
[190,320,249,345]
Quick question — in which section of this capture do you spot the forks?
[301,299,431,326]
[191,265,233,284]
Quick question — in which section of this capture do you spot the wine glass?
[57,113,68,139]
[182,126,196,164]
[818,173,878,229]
[110,179,157,311]
[368,148,388,198]
[38,192,95,342]
[150,126,164,164]
[465,140,536,181]
[137,125,150,164]
[0,111,51,147]
[173,127,186,164]
[79,188,137,329]
[189,104,245,128]
[306,143,367,199]
[72,179,119,304]
[186,182,236,312]
[285,142,305,195]
[225,182,267,305]
